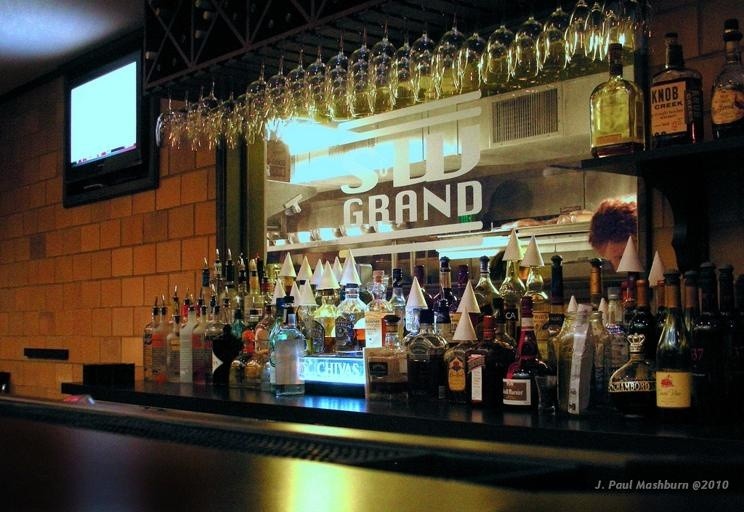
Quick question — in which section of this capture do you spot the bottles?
[140,255,743,428]
[590,27,741,158]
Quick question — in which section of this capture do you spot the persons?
[587,198,638,275]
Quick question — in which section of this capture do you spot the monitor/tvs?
[65,49,143,185]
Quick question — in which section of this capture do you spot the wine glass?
[155,0,646,153]
